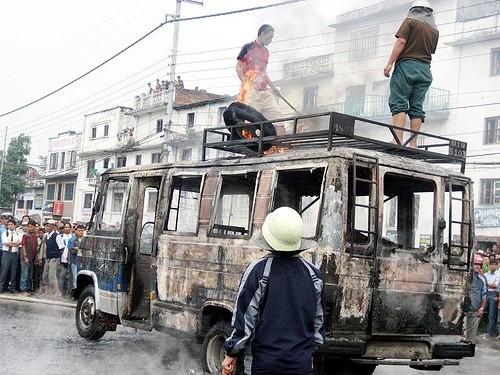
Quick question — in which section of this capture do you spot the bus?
[71,113,476,375]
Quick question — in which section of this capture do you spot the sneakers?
[38,293,49,298]
[52,292,62,298]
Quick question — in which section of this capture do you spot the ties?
[9,232,15,254]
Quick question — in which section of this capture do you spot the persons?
[466,253,489,344]
[147,76,184,92]
[383,0,440,148]
[235,24,287,136]
[483,258,500,338]
[220,206,327,375]
[0,208,107,301]
[475,245,500,265]
[195,86,198,91]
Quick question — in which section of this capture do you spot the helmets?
[262,207,303,251]
[474,254,483,264]
[409,0,433,12]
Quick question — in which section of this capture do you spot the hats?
[58,223,64,229]
[22,222,28,225]
[45,220,56,226]
